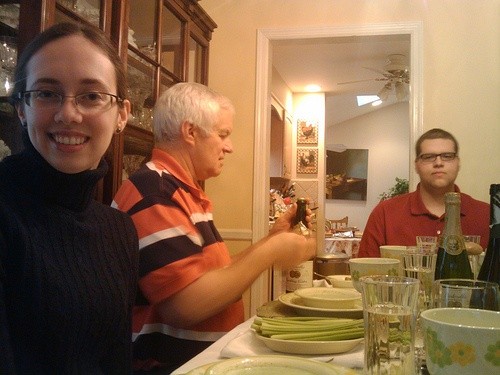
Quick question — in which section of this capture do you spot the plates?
[278,293,364,319]
[255,332,365,354]
[186,355,358,375]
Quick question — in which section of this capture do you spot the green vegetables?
[251,317,365,340]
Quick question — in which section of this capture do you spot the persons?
[353,127,491,259]
[0,19,141,375]
[109,83,316,375]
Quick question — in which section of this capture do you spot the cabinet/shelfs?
[0,0,218,208]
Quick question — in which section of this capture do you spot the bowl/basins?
[293,287,364,308]
[206,356,338,375]
[327,275,353,287]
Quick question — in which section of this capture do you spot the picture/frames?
[297,117,319,144]
[296,148,318,175]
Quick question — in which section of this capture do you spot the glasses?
[22,89,122,114]
[418,151,459,162]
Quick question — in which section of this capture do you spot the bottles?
[434,191,474,307]
[468,184,500,311]
[286,197,313,294]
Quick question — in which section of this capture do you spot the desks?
[318,232,363,279]
[169,279,424,375]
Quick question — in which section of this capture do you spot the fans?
[336,54,410,86]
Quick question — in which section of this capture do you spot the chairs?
[328,216,349,231]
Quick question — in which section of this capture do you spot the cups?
[349,234,500,375]
[0,0,157,177]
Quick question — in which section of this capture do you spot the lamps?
[376,80,409,102]
